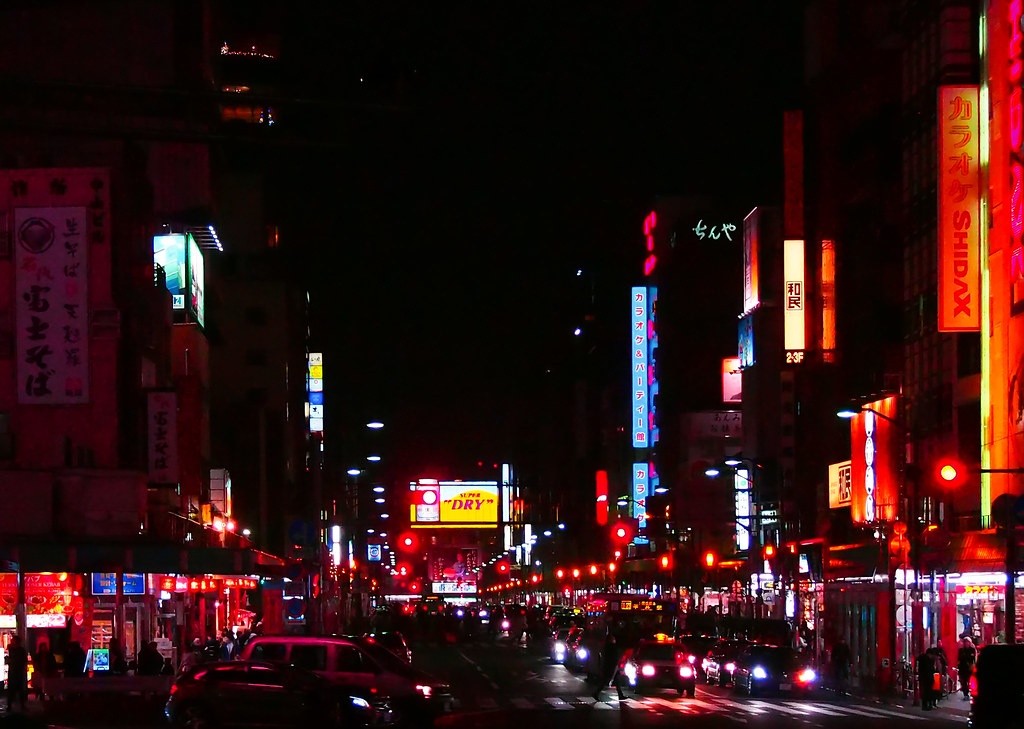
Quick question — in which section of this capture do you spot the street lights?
[834,404,930,700]
[616,498,677,589]
[706,457,764,621]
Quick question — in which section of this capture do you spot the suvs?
[166,632,455,729]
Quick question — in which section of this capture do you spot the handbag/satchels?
[932,672,942,691]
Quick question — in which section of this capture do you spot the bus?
[585,592,682,680]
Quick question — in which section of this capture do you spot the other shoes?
[593,693,602,702]
[6,706,11,712]
[922,705,943,711]
[964,696,970,700]
[619,696,628,700]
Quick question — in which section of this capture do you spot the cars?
[613,633,696,698]
[463,601,585,664]
[683,632,819,698]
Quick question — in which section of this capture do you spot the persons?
[592,631,630,701]
[832,638,850,696]
[31,642,59,701]
[191,626,263,658]
[916,647,939,711]
[57,637,85,678]
[958,632,977,701]
[5,636,27,712]
[137,640,175,678]
